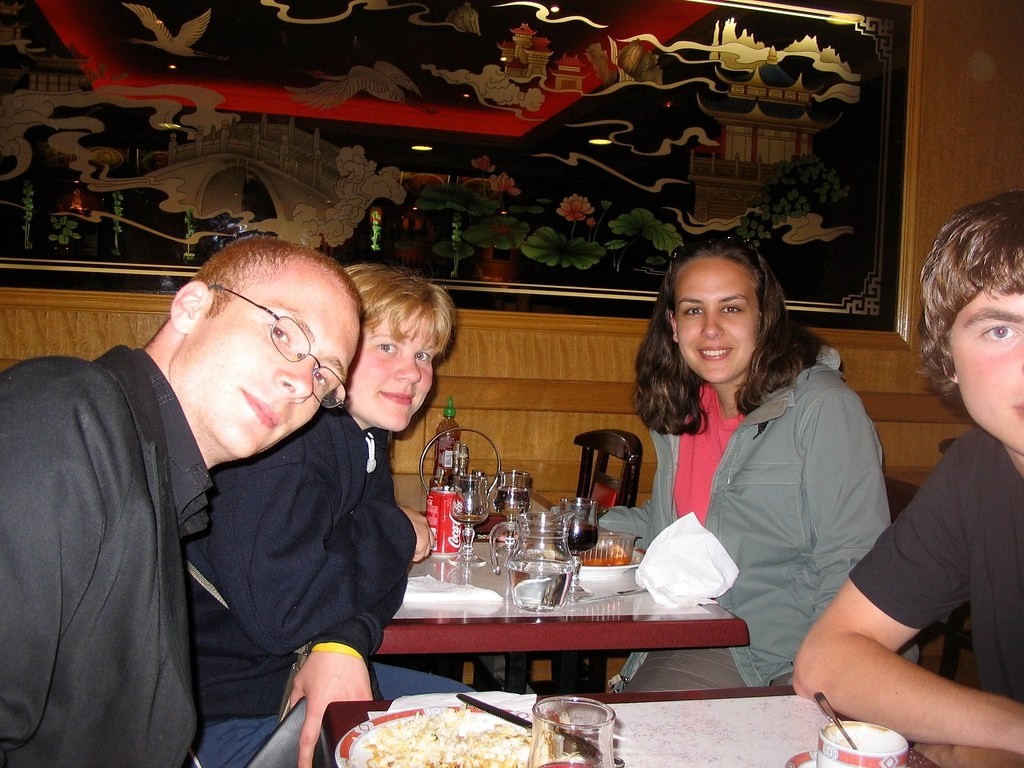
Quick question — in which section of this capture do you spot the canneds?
[424,484,464,555]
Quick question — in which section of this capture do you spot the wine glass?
[553,497,599,598]
[493,470,530,556]
[448,474,489,567]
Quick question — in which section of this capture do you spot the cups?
[816,721,909,768]
[525,695,617,768]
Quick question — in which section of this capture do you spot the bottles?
[433,396,461,486]
[447,442,475,493]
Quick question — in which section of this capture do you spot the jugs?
[488,510,580,613]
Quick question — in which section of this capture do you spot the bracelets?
[313,643,362,656]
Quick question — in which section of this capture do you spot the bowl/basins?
[578,531,635,567]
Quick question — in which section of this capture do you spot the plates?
[578,550,644,580]
[785,750,818,768]
[333,704,599,768]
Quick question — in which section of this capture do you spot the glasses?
[206,284,347,411]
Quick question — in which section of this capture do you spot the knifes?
[456,693,625,768]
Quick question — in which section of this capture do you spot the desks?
[323,676,936,768]
[376,469,753,693]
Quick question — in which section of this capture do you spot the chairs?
[476,431,647,695]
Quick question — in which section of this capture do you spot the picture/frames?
[0,0,927,349]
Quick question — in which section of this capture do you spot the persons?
[607,229,918,692]
[185,263,473,768]
[0,237,363,768]
[791,191,1024,768]
